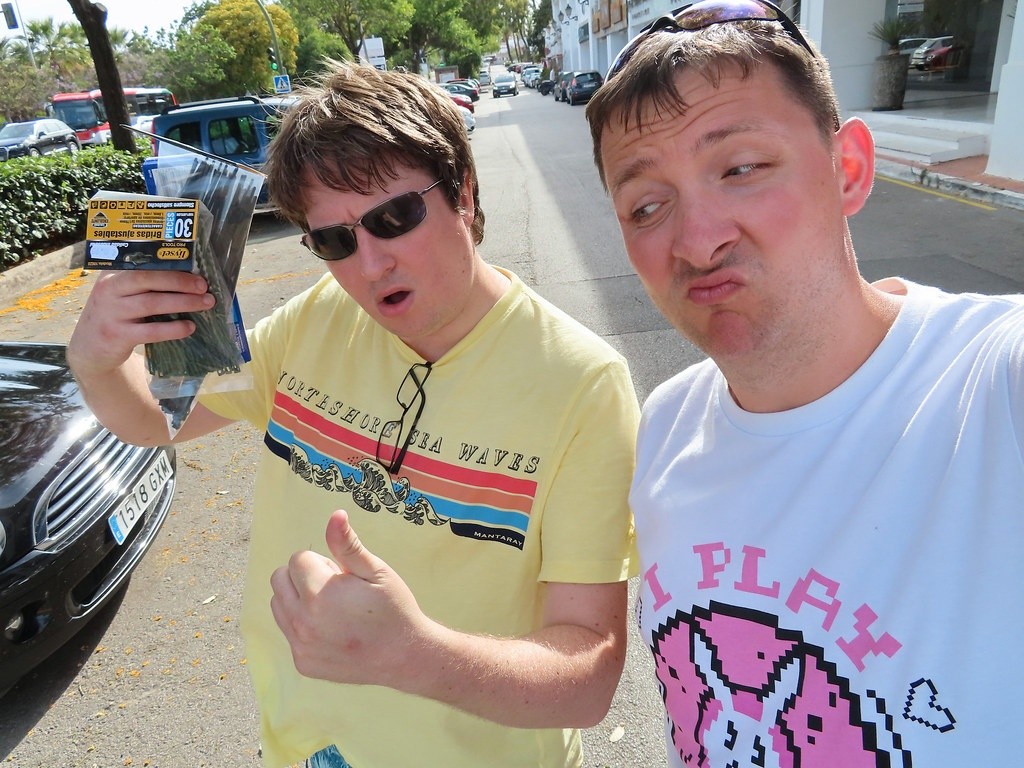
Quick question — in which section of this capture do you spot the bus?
[45,87,177,145]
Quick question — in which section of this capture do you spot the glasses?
[603,0,822,84]
[300,178,445,261]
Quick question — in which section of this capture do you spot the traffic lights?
[266,47,278,70]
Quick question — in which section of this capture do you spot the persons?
[66,60,641,767]
[586,0,1024,767]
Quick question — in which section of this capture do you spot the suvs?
[552,71,571,102]
[565,71,603,106]
[152,94,302,216]
[493,73,519,98]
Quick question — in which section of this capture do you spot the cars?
[479,64,491,86]
[0,342,178,696]
[437,78,480,131]
[507,62,552,94]
[0,119,82,161]
